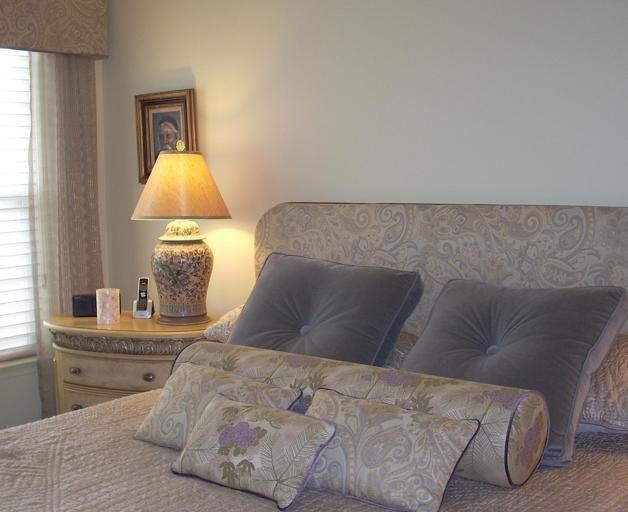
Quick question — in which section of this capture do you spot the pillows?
[170,391,336,512]
[169,340,551,491]
[133,361,301,453]
[224,252,423,369]
[301,385,480,512]
[399,278,627,472]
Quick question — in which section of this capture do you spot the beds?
[0,203,628,512]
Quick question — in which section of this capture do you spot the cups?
[96,288,120,325]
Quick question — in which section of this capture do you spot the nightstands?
[44,313,223,417]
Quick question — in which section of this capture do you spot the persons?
[155,116,181,154]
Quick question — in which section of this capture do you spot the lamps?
[131,149,231,325]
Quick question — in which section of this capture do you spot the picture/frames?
[133,89,197,186]
